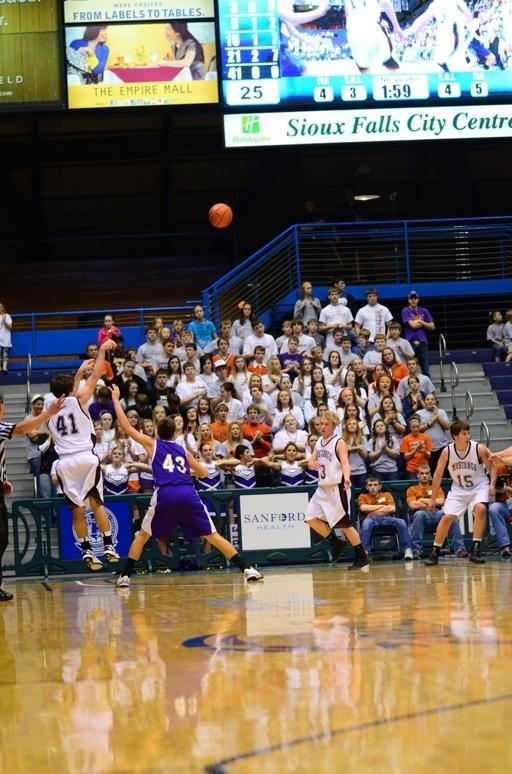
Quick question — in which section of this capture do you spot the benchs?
[477,343,512,423]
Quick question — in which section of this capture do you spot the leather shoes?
[0,589,14,601]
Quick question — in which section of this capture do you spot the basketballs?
[209,202,233,228]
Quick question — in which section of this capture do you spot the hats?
[408,290,420,299]
[213,359,227,369]
[30,393,44,404]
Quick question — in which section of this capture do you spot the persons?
[280,0,512,78]
[0,280,512,600]
[300,189,380,284]
[158,22,207,80]
[68,24,112,85]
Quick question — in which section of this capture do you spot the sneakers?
[500,547,511,560]
[457,548,468,559]
[470,546,486,563]
[348,547,371,570]
[404,549,421,561]
[244,566,264,581]
[425,548,440,566]
[330,539,348,565]
[104,546,121,565]
[82,550,104,571]
[116,575,131,587]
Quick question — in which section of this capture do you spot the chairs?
[351,494,512,564]
